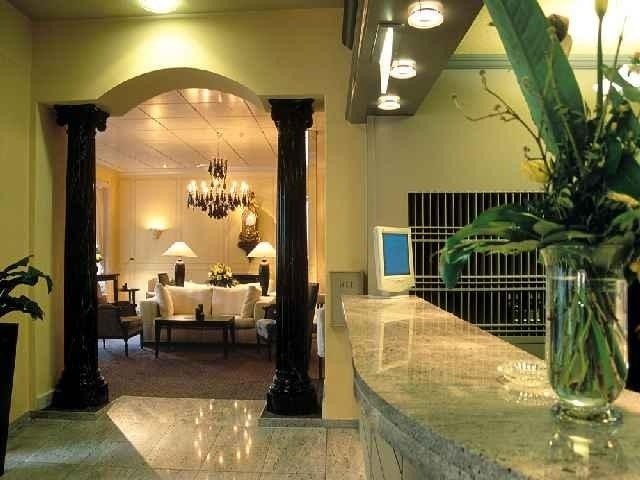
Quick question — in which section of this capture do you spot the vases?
[539,242,629,429]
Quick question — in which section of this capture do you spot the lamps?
[150,228,166,240]
[244,239,278,297]
[407,1,448,31]
[161,238,196,287]
[376,89,403,115]
[392,55,421,81]
[184,123,254,220]
[232,202,262,257]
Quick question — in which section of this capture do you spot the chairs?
[95,300,143,352]
[251,281,320,360]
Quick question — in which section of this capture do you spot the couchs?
[140,283,270,346]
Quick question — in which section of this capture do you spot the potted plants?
[0,251,54,478]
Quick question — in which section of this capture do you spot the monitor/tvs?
[372,226,418,296]
[371,301,415,374]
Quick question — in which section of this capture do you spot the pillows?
[154,283,175,318]
[236,284,263,319]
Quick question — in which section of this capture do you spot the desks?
[118,287,141,304]
[232,272,260,284]
[95,272,120,304]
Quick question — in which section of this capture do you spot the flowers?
[206,261,238,288]
[435,0,640,404]
